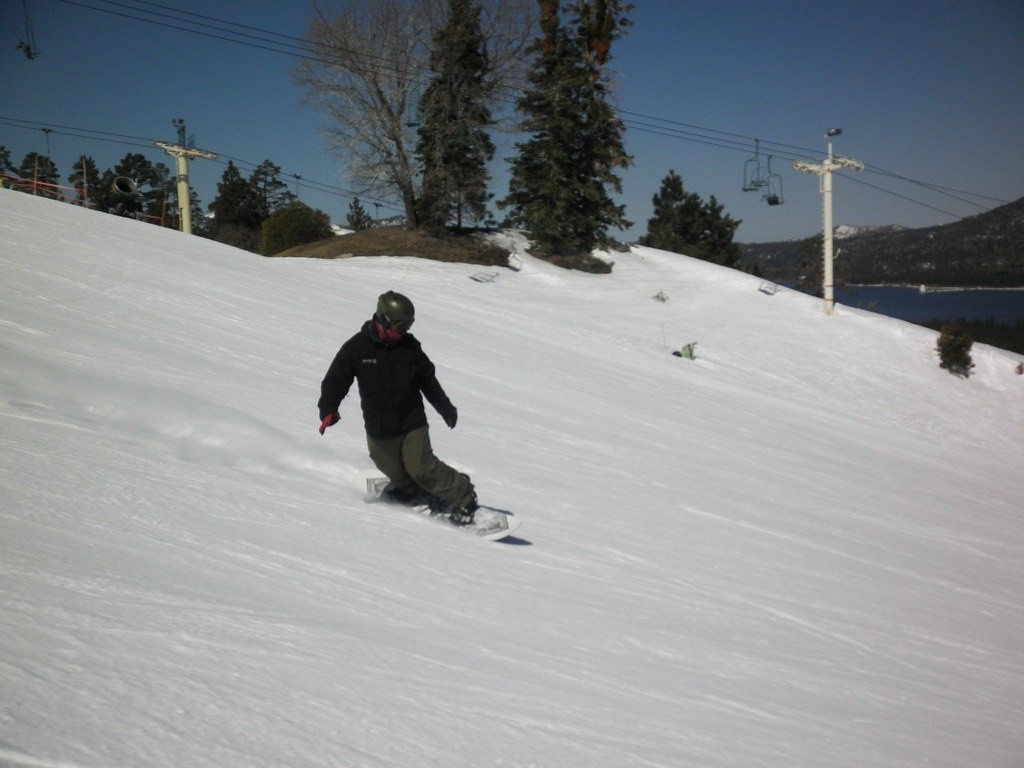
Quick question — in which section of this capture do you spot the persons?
[317,290,479,526]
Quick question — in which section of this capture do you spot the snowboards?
[366,477,521,542]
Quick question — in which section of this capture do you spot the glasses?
[389,320,409,333]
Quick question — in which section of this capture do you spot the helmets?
[377,290,414,326]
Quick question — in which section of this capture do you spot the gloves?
[320,413,342,435]
[441,405,457,428]
